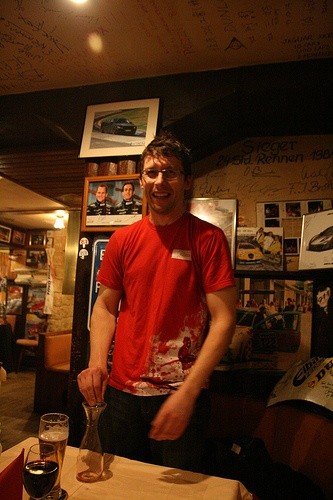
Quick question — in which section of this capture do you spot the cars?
[100,117,137,135]
[208,306,302,364]
[235,243,262,265]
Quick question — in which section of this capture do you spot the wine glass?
[22,443,59,500]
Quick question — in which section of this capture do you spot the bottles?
[73,401,108,482]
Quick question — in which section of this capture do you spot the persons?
[113,182,142,215]
[256,227,281,258]
[239,296,312,332]
[76,133,239,477]
[86,183,116,216]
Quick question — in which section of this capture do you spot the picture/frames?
[296,208,333,272]
[235,226,285,274]
[186,197,239,271]
[207,274,320,379]
[254,199,333,257]
[0,221,54,366]
[79,173,148,232]
[74,95,162,159]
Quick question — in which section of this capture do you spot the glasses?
[139,167,187,178]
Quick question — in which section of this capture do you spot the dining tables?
[0,435,253,500]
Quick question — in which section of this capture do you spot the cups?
[37,413,69,500]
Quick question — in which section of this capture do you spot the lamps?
[53,210,67,230]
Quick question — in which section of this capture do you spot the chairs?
[249,355,332,500]
